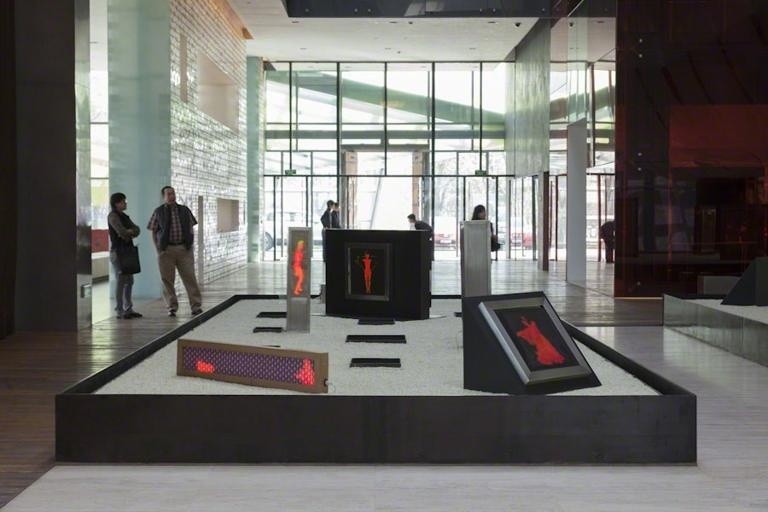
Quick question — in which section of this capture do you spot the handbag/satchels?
[115,236,141,276]
[490,222,500,252]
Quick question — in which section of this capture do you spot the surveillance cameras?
[568,23,574,27]
[513,23,520,27]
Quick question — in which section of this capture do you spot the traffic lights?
[178,345,315,385]
[348,246,386,297]
[287,227,310,298]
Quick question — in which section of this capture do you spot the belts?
[168,242,186,246]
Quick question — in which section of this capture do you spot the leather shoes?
[168,310,177,317]
[116,311,143,320]
[192,308,203,315]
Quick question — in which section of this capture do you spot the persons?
[147,185,203,317]
[107,192,145,321]
[407,212,434,241]
[597,218,615,265]
[331,201,341,228]
[470,204,498,253]
[318,200,336,264]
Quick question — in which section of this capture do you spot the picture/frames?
[343,241,393,302]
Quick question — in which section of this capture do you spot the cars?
[434,216,460,247]
[490,220,533,247]
[262,211,323,251]
